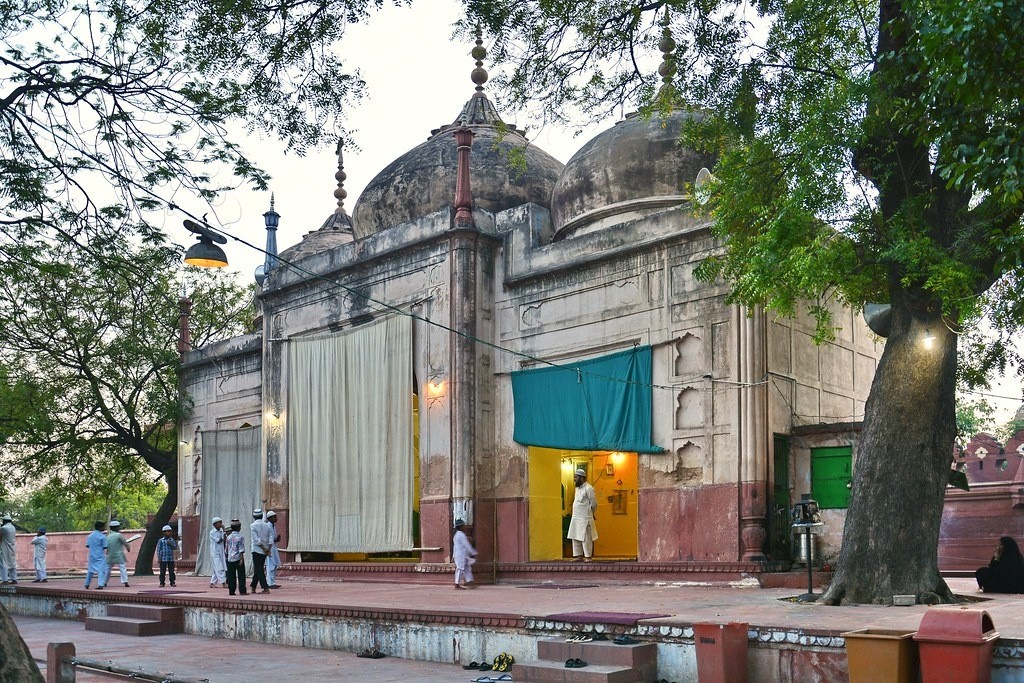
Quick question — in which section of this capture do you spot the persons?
[0,509,283,595]
[567,468,599,563]
[452,519,480,590]
[976,537,1024,594]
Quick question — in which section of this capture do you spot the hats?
[212,517,222,523]
[455,520,465,526]
[575,469,586,477]
[109,521,120,526]
[2,516,12,520]
[231,518,241,525]
[38,527,46,533]
[267,511,276,518]
[162,525,172,532]
[253,508,263,516]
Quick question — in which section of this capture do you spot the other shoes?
[662,679,667,683]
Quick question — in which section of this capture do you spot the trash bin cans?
[839,629,917,683]
[691,621,749,683]
[792,533,817,563]
[912,609,1001,683]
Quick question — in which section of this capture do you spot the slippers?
[470,674,513,683]
[573,635,593,642]
[478,662,494,671]
[357,650,386,658]
[565,659,574,667]
[573,658,588,667]
[492,652,512,672]
[566,635,580,642]
[463,662,481,670]
[613,635,639,644]
